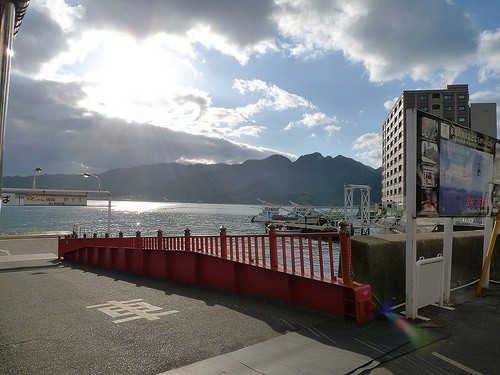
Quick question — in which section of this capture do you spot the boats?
[252,206,340,243]
